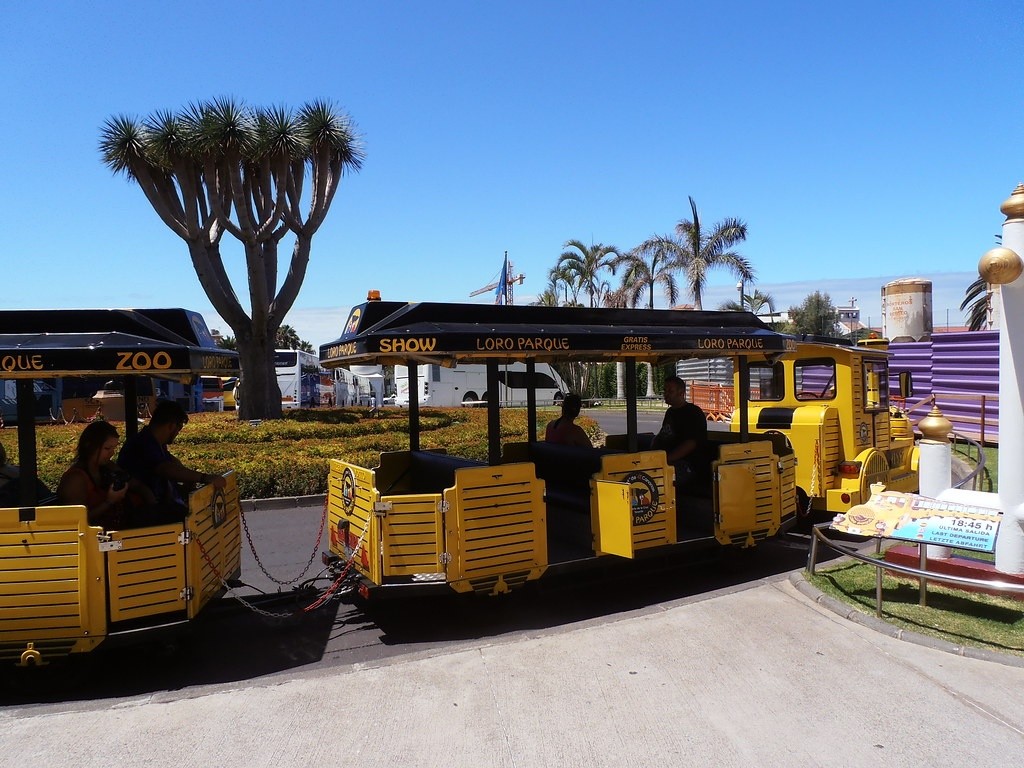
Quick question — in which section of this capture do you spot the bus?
[275,348,371,410]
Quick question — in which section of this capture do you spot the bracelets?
[104,500,113,508]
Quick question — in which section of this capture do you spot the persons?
[120,400,226,521]
[60,421,156,528]
[546,396,594,450]
[649,376,708,495]
[0,443,49,500]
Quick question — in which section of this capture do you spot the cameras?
[104,470,132,491]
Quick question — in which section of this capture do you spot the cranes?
[469,260,526,306]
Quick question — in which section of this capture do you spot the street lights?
[736,278,744,307]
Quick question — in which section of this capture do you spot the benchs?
[638,433,746,531]
[528,439,630,543]
[410,450,488,493]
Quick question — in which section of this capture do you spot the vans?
[201,375,240,413]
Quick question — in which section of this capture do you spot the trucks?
[392,363,570,408]
